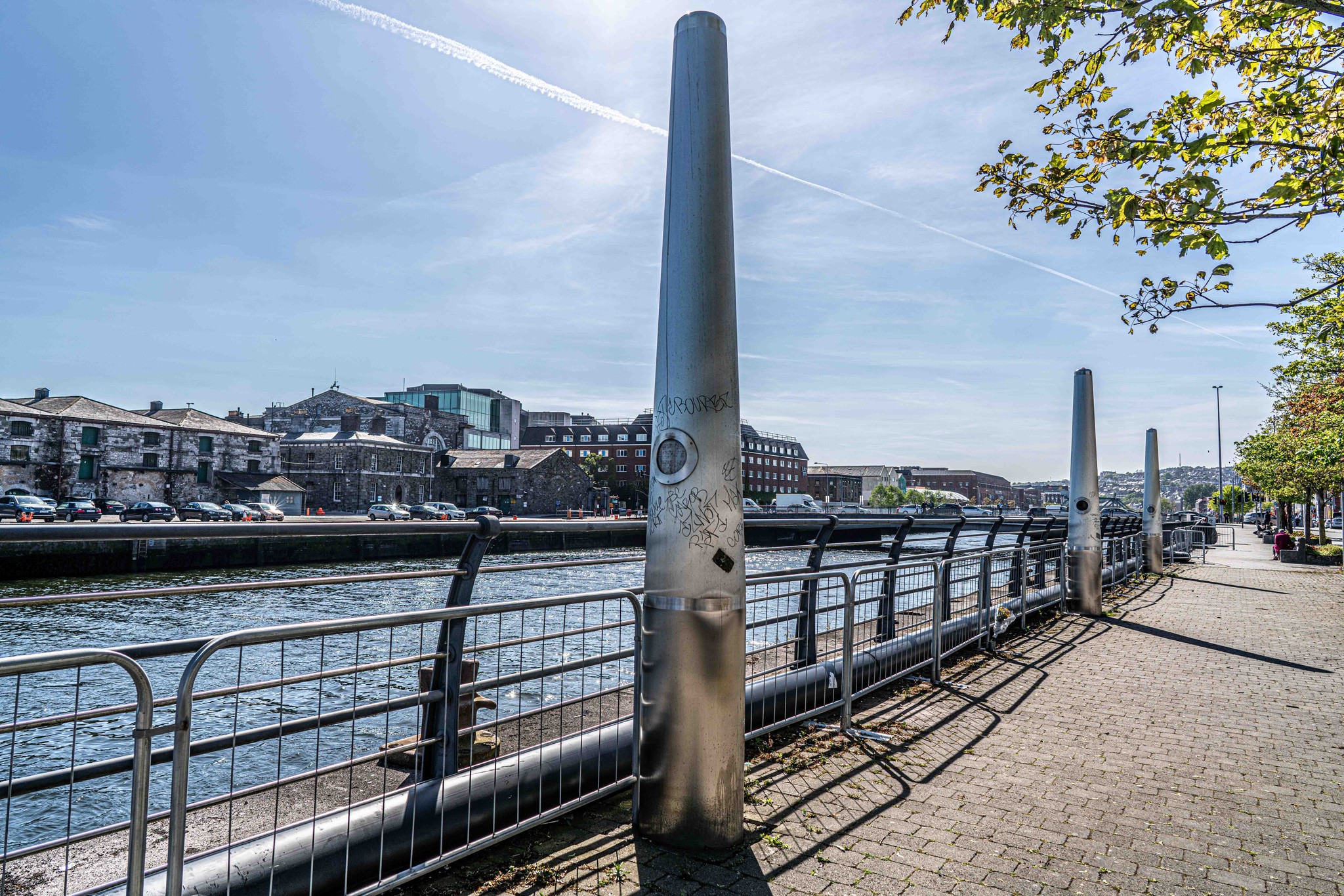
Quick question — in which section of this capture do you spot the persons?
[1272,529,1291,560]
[225,500,230,504]
[1223,511,1230,524]
[929,500,934,514]
[1257,523,1266,531]
[1235,513,1238,517]
[1264,511,1271,524]
[747,503,751,506]
[914,502,919,514]
[998,501,1003,515]
[1310,512,1316,527]
[1284,511,1288,531]
[1295,513,1300,527]
[921,500,929,514]
[771,500,779,512]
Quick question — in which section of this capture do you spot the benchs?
[1263,529,1277,544]
[1279,536,1306,564]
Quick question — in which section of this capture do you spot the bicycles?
[919,508,932,514]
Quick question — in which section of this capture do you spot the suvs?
[1242,511,1277,525]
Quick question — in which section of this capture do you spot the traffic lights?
[638,493,642,500]
[1312,508,1317,514]
[1212,496,1226,505]
[636,470,640,477]
[1329,496,1333,502]
[1244,491,1257,502]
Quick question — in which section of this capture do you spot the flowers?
[995,606,1010,624]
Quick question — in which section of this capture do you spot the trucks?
[742,498,763,513]
[775,493,822,513]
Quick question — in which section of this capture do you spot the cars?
[838,505,871,514]
[786,504,817,513]
[896,504,924,514]
[1027,506,1046,517]
[934,503,993,516]
[465,506,503,521]
[0,494,285,524]
[1099,507,1141,519]
[1166,511,1209,522]
[367,504,446,521]
[1002,511,1028,516]
[1312,517,1342,529]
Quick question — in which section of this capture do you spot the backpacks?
[914,504,916,508]
[930,503,933,506]
[997,504,1000,508]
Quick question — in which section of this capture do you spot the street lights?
[814,462,828,514]
[848,468,862,505]
[960,486,1018,508]
[1212,385,1224,523]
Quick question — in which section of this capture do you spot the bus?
[597,495,620,511]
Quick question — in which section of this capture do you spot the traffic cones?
[241,509,248,522]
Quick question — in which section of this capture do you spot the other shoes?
[1271,556,1279,560]
[1296,526,1299,527]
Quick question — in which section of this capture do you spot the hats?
[1226,511,1227,512]
[1278,529,1284,532]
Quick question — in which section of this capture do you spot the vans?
[1045,505,1069,518]
[1333,512,1342,518]
[425,502,466,521]
